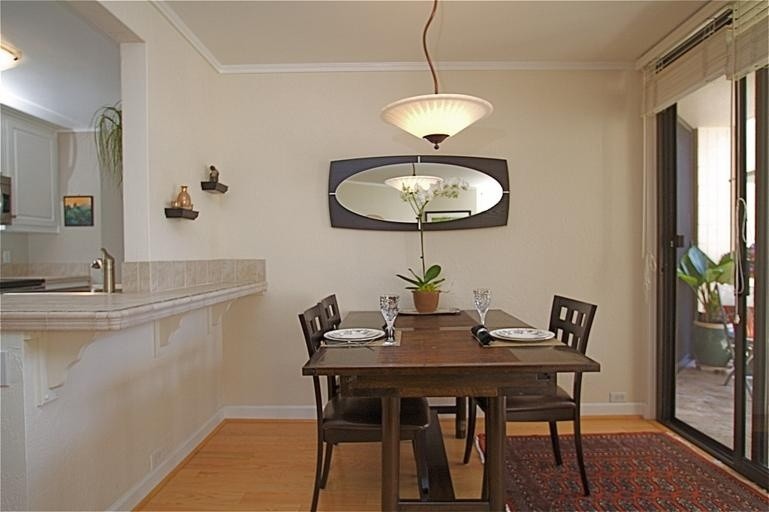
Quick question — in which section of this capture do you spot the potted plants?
[677,248,737,375]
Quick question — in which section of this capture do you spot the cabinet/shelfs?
[0,110,59,235]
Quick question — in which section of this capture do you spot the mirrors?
[326,154,510,233]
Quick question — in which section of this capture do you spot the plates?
[324,328,386,341]
[489,327,555,343]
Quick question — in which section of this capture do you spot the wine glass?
[474,288,492,325]
[380,294,399,345]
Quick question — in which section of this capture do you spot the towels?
[470,324,493,345]
[383,322,394,340]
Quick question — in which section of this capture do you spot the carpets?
[471,432,769,512]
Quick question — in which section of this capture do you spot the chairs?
[298,305,432,510]
[463,294,599,501]
[320,294,346,392]
[426,396,468,440]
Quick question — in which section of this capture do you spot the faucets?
[88,247,117,295]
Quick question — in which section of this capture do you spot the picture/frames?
[62,194,93,227]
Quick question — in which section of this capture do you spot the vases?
[409,289,443,313]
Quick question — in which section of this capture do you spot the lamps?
[383,164,444,193]
[378,2,494,153]
[0,36,20,75]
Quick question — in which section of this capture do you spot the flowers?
[401,176,472,221]
[395,177,471,294]
